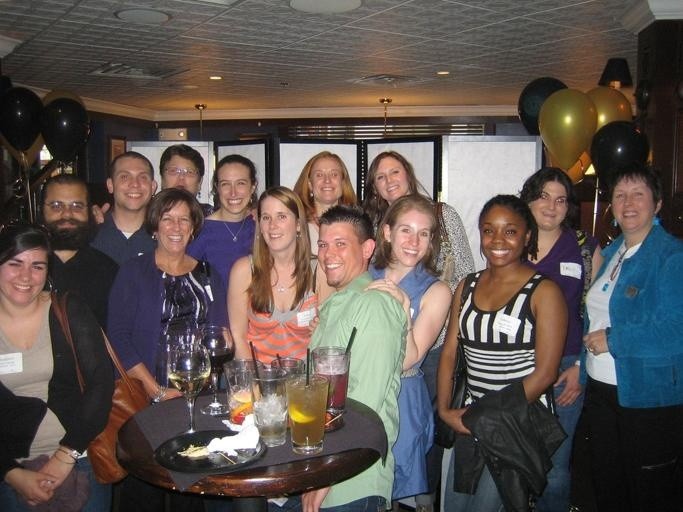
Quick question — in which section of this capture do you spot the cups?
[223,346,349,456]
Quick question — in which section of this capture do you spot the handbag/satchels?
[87,378,152,483]
[431,337,467,449]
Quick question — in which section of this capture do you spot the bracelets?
[152,385,171,405]
[573,355,586,371]
[48,442,80,466]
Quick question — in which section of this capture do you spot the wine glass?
[165,325,235,434]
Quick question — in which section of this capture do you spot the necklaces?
[271,258,292,292]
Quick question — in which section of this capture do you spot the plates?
[151,430,267,472]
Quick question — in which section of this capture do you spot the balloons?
[2,84,93,202]
[516,73,654,189]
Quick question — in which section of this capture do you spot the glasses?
[164,167,201,177]
[46,202,88,210]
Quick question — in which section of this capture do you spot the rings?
[46,478,55,485]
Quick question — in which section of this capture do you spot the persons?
[367,195,452,512]
[2,219,112,512]
[29,171,121,332]
[187,156,266,337]
[158,144,216,217]
[90,151,166,268]
[364,151,475,511]
[105,186,230,406]
[293,149,362,279]
[519,166,604,511]
[436,194,568,512]
[298,204,410,511]
[227,186,338,399]
[569,168,681,511]
